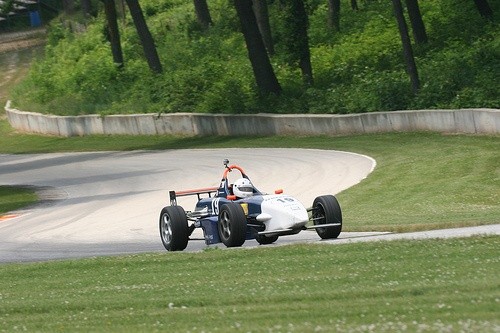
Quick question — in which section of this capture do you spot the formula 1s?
[158,158,344,252]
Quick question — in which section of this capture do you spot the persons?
[232,177,253,199]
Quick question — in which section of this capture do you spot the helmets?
[233,178,254,199]
